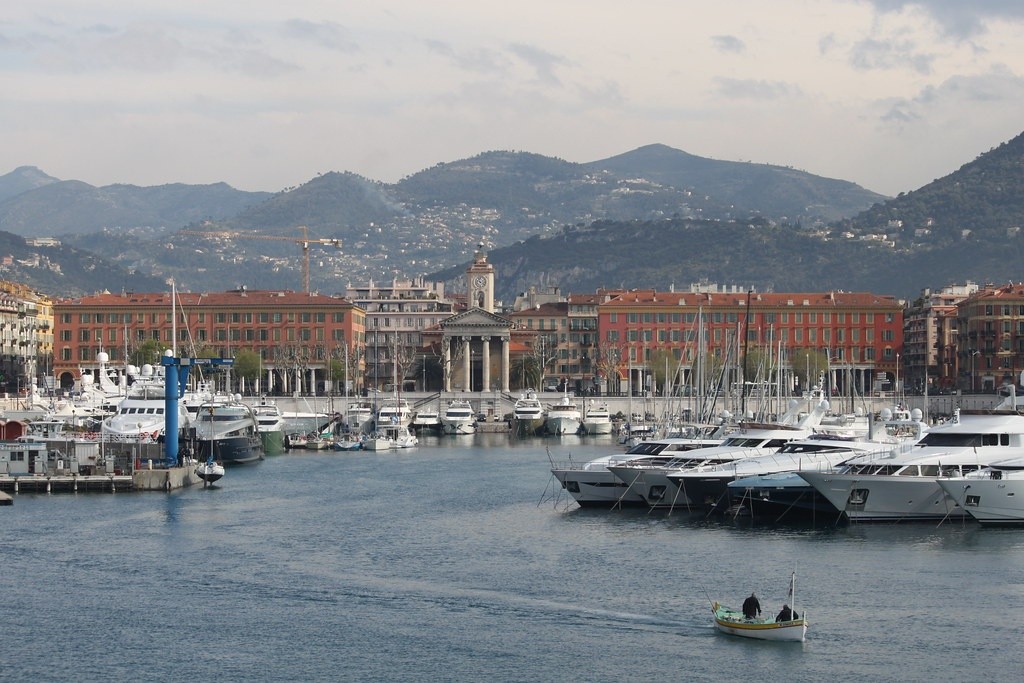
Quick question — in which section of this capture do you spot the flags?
[788,579,793,597]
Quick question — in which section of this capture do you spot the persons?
[184,446,191,466]
[743,592,762,619]
[775,604,799,623]
[177,448,184,467]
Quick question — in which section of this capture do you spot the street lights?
[971,349,980,394]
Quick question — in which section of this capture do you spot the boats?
[510,387,544,437]
[23,275,419,483]
[546,378,584,437]
[582,399,613,434]
[710,570,809,643]
[439,399,475,435]
[412,412,442,429]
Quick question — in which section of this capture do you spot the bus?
[730,380,778,398]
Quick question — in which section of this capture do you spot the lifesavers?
[79,432,88,442]
[153,430,159,439]
[144,432,149,437]
[138,433,145,440]
[87,432,96,440]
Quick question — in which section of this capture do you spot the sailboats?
[551,300,1024,530]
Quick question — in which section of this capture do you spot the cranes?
[177,225,344,296]
